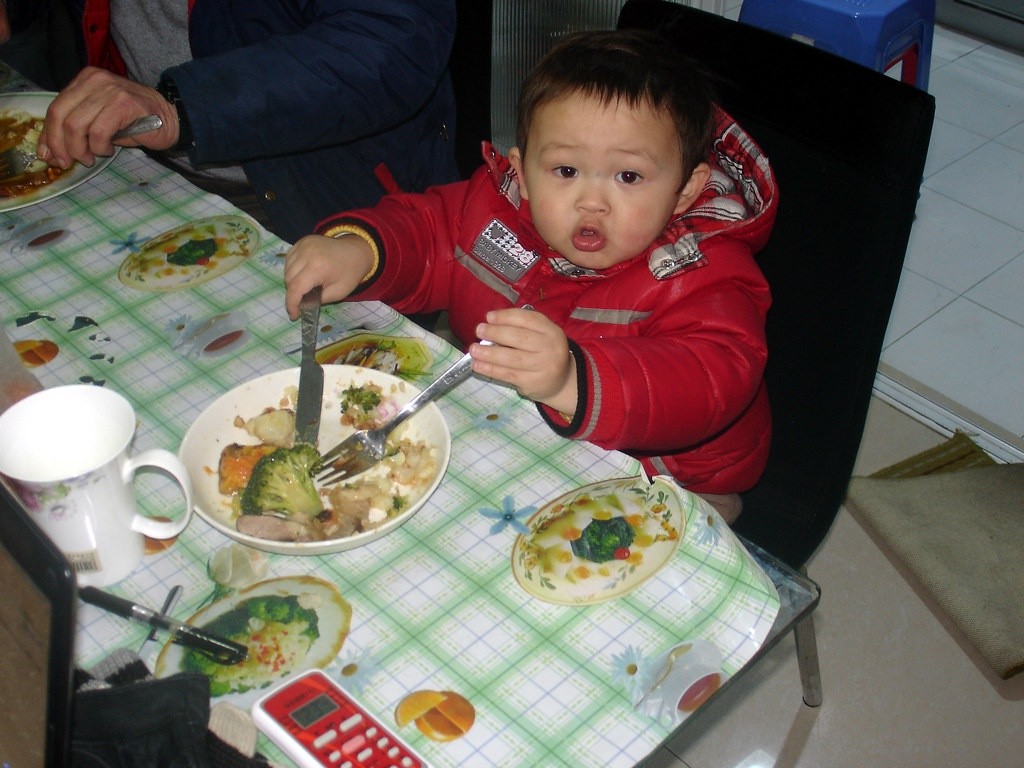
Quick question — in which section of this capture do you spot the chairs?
[0,482,235,768]
[613,0,935,576]
[407,0,494,333]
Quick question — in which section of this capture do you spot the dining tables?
[0,90,821,768]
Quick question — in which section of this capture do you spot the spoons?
[173,313,231,353]
[632,643,693,711]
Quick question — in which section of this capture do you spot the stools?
[737,0,934,93]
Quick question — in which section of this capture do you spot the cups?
[185,321,255,362]
[0,385,194,588]
[11,226,72,257]
[656,658,727,729]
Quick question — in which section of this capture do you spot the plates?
[180,364,451,553]
[117,214,261,291]
[652,638,722,693]
[153,575,353,714]
[10,215,71,242]
[316,332,431,376]
[511,476,687,606]
[183,311,248,349]
[0,85,121,214]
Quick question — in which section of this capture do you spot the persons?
[286,30,779,524]
[0,0,460,333]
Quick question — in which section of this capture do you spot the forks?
[0,115,163,181]
[311,305,532,487]
[283,309,397,354]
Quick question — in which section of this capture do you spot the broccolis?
[242,387,386,522]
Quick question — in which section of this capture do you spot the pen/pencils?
[75,584,248,667]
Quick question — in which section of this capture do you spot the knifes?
[293,287,324,448]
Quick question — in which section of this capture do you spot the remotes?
[251,667,433,768]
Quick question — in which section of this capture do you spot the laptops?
[0,476,81,768]
